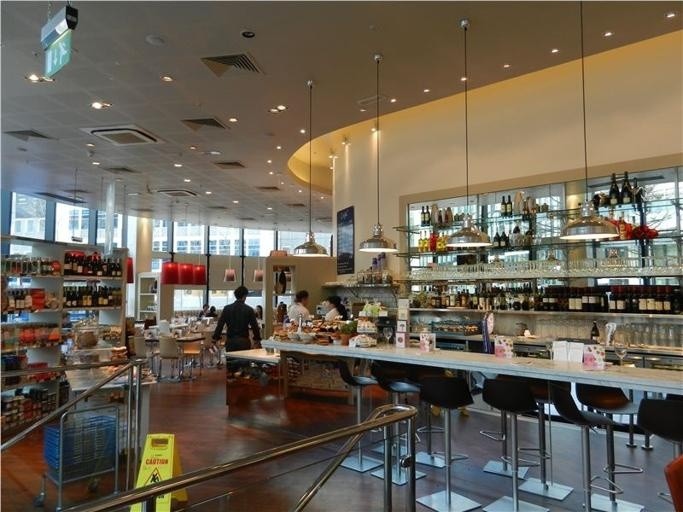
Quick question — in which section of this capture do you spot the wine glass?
[612,349,628,373]
[382,327,394,348]
[406,255,681,280]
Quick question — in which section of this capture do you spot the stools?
[337,354,682,511]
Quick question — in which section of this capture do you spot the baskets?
[42,413,118,475]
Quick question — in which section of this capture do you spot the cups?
[614,323,683,348]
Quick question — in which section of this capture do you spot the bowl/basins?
[287,332,316,345]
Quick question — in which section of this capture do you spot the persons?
[254,305,262,319]
[325,295,348,322]
[199,304,209,318]
[287,290,311,326]
[205,306,218,318]
[212,286,262,351]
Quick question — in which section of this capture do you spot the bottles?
[273,270,291,294]
[409,282,682,313]
[493,225,541,248]
[593,214,638,242]
[412,320,479,333]
[418,203,468,252]
[6,289,34,312]
[0,251,61,275]
[589,321,600,343]
[1,321,61,350]
[501,194,512,216]
[513,192,549,214]
[611,171,641,206]
[63,252,122,308]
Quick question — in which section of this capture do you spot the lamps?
[442,16,493,249]
[554,2,620,242]
[125,183,264,286]
[290,77,331,258]
[356,52,400,254]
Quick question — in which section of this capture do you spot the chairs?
[127,313,266,383]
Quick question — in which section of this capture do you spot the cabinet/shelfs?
[0,232,130,436]
[266,257,298,340]
[392,195,682,281]
